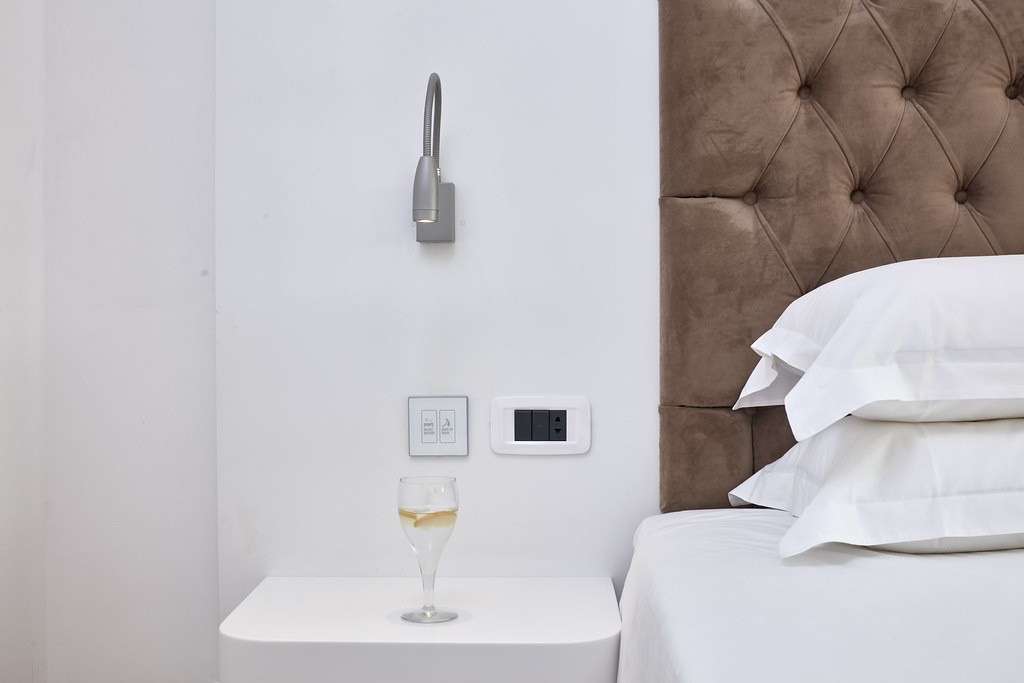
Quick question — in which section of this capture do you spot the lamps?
[412,72,443,224]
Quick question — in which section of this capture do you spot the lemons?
[413,510,456,528]
[399,509,416,521]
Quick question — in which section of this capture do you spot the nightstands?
[218,575,622,683]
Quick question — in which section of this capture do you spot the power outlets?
[416,183,458,243]
[490,395,595,457]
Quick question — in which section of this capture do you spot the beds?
[615,0,1024,683]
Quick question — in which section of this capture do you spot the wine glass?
[399,477,458,623]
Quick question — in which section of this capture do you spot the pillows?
[727,416,1024,558]
[735,252,1024,443]
